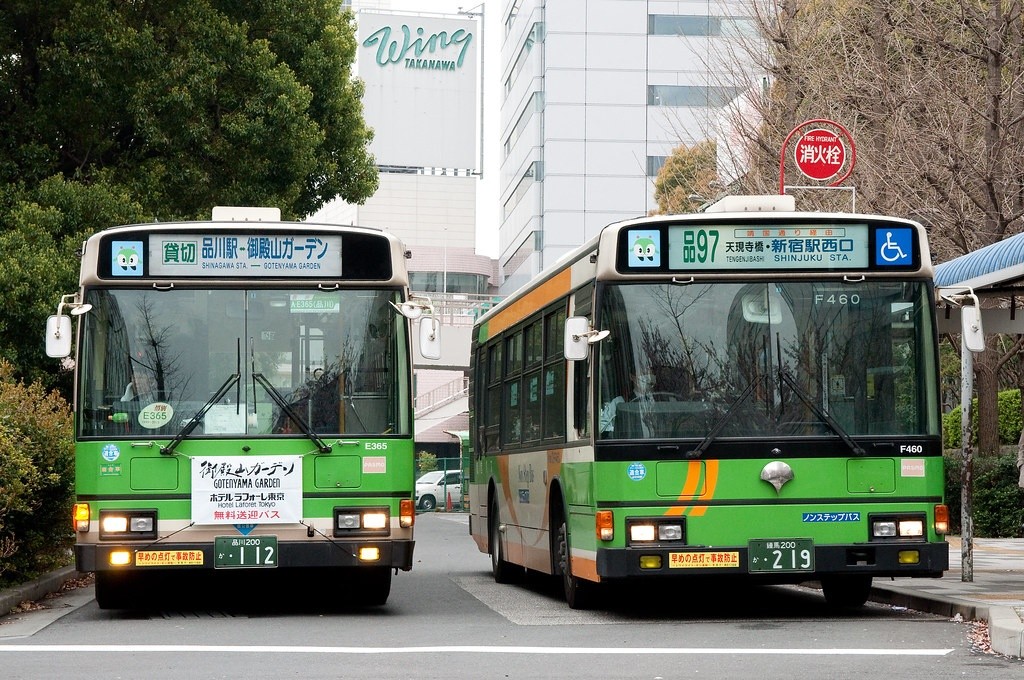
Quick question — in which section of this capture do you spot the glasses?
[129,376,149,381]
[636,370,656,375]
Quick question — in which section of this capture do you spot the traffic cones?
[447,493,454,512]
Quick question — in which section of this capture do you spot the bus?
[44,206,441,612]
[469,196,984,608]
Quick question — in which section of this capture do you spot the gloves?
[120,381,134,402]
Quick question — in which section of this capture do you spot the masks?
[635,375,657,390]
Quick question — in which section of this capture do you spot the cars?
[416,470,463,512]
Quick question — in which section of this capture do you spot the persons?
[121,361,171,403]
[600,359,677,442]
